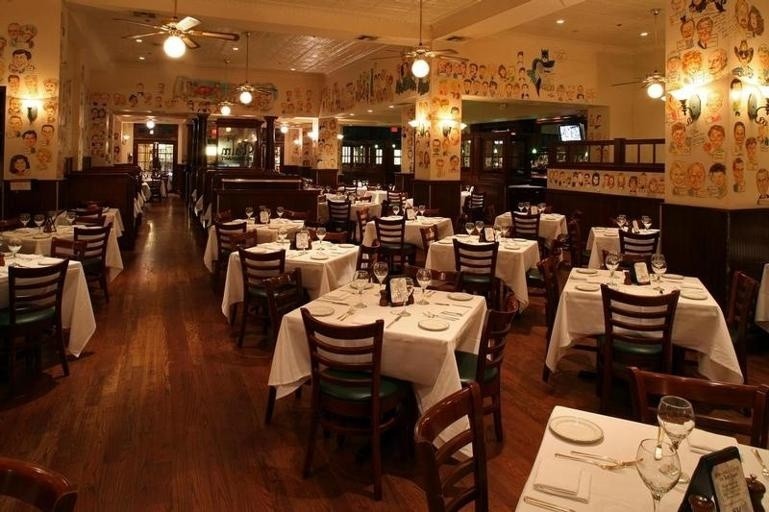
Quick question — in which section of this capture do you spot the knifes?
[385,316,401,329]
[652,426,665,461]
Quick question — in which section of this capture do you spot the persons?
[546,168,665,199]
[400,95,461,178]
[127,77,315,114]
[319,52,430,116]
[86,92,126,164]
[0,20,58,181]
[285,117,339,169]
[665,0,768,209]
[436,50,600,104]
[588,111,610,161]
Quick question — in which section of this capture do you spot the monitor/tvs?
[557,122,585,144]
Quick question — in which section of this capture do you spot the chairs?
[147,176,169,202]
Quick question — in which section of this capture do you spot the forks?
[552,450,644,471]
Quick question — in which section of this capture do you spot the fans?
[114,1,240,50]
[367,1,467,62]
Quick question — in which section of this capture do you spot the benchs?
[190,169,320,234]
[58,162,143,250]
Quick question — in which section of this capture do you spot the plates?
[576,268,598,274]
[308,243,355,262]
[308,306,335,316]
[549,416,605,444]
[575,283,602,292]
[679,289,709,300]
[505,245,521,250]
[439,240,453,244]
[448,292,473,300]
[663,274,684,280]
[418,319,451,331]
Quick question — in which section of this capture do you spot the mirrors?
[206,121,258,169]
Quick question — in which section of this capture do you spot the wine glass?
[396,277,414,317]
[277,227,327,255]
[352,178,393,190]
[616,214,653,233]
[517,201,546,217]
[393,204,426,223]
[19,213,45,235]
[463,220,510,245]
[1,236,23,259]
[635,395,697,511]
[373,259,432,306]
[245,205,285,226]
[354,271,369,309]
[604,248,667,293]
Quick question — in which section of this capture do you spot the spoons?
[752,448,769,480]
